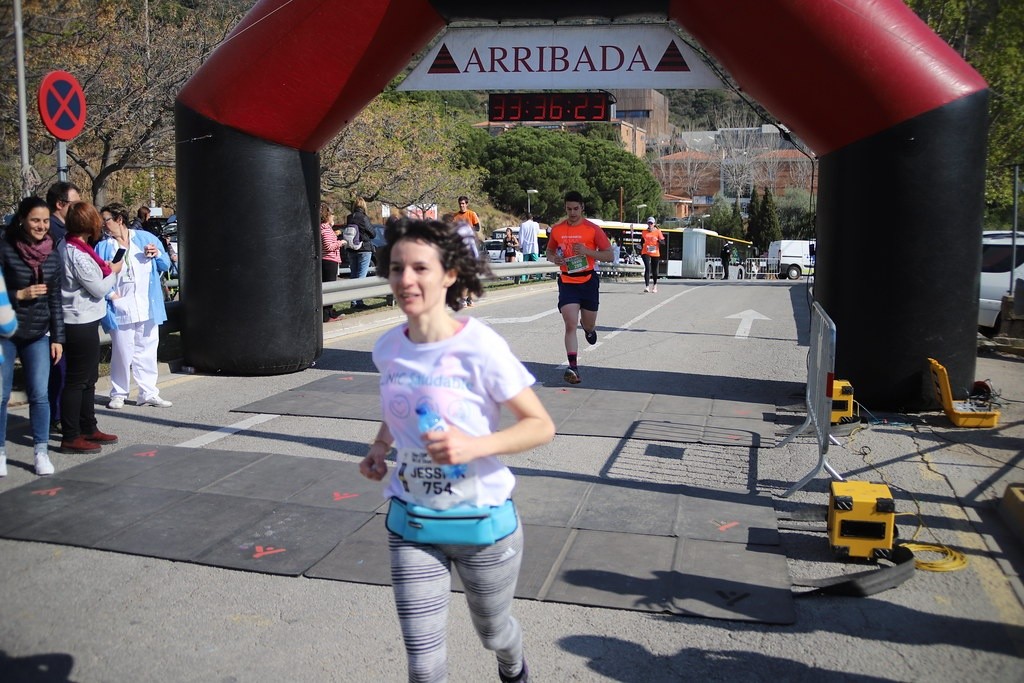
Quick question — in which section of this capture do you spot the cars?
[162,222,178,255]
[331,224,388,277]
[483,240,523,264]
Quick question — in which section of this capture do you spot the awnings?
[717,235,753,246]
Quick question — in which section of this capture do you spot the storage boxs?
[928,357,1000,427]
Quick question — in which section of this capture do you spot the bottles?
[556,246,566,265]
[637,245,642,249]
[414,403,469,480]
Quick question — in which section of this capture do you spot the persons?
[502,228,519,262]
[55,201,118,453]
[45,182,82,434]
[132,206,151,230]
[636,217,665,293]
[94,202,172,408]
[346,197,376,308]
[359,217,555,683]
[0,196,64,476]
[0,268,19,406]
[546,191,615,383]
[442,196,479,309]
[519,213,541,282]
[321,202,347,322]
[720,241,730,280]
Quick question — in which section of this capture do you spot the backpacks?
[343,214,366,252]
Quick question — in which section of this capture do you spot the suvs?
[978,230,1024,338]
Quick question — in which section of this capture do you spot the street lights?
[636,204,647,223]
[528,189,538,213]
[701,214,711,229]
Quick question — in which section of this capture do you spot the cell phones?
[37,267,44,284]
[112,248,126,264]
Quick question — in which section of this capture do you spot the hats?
[647,217,656,224]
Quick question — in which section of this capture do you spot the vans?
[767,240,816,280]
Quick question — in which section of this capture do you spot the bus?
[586,219,649,264]
[660,228,760,279]
[493,228,548,262]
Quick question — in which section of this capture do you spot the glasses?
[103,217,114,224]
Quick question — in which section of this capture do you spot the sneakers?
[137,396,173,407]
[0,455,7,477]
[564,367,582,383]
[109,398,125,408]
[60,436,102,453]
[580,320,597,345]
[80,427,119,444]
[324,309,346,322]
[33,451,55,475]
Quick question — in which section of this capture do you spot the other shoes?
[467,299,473,305]
[355,305,370,310]
[498,656,528,683]
[652,288,657,292]
[644,287,650,292]
[49,420,63,436]
[460,299,467,307]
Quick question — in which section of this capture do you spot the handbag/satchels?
[386,497,517,546]
[507,247,514,253]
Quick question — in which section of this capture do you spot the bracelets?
[375,440,389,452]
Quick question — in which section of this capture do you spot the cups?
[143,245,152,258]
